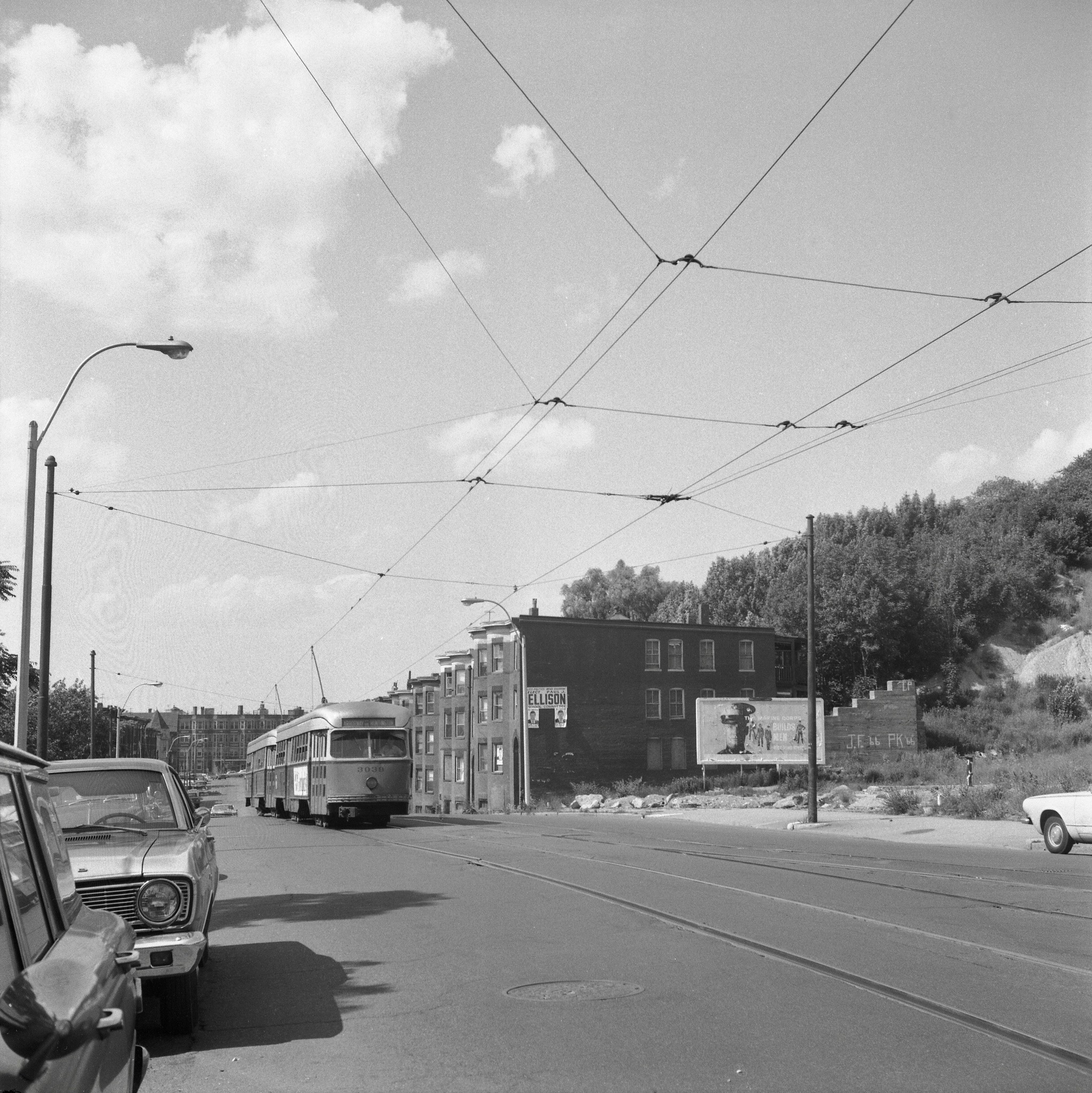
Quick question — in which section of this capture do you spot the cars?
[1022,782,1092,854]
[0,740,150,1093]
[46,758,217,1035]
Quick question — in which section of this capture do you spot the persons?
[748,719,757,741]
[496,747,503,761]
[795,720,806,745]
[755,723,765,748]
[527,710,539,725]
[555,709,567,724]
[765,726,773,751]
[379,737,404,757]
[715,702,754,756]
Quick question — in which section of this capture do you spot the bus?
[246,647,413,829]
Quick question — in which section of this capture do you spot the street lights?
[115,681,162,759]
[13,336,193,751]
[167,736,191,765]
[186,738,208,776]
[461,598,531,806]
